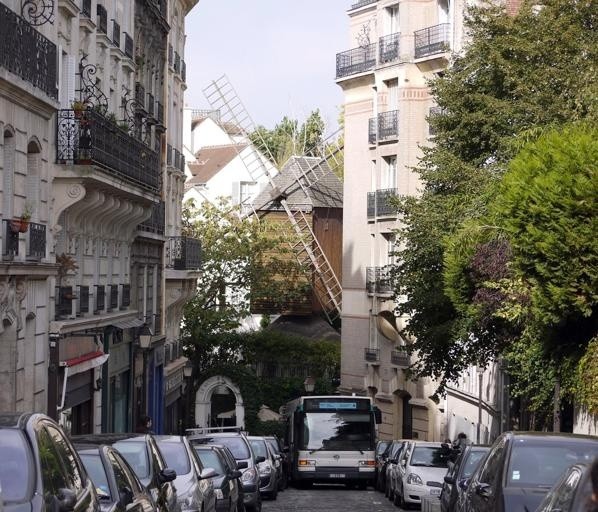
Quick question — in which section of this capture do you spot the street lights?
[136,321,152,417]
[181,359,194,436]
[302,375,316,395]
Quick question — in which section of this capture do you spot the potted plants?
[72,101,85,118]
[19,200,35,232]
[80,153,91,164]
[79,118,95,144]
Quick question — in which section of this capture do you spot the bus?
[277,394,385,494]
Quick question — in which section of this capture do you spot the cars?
[371,436,493,512]
[0,407,287,511]
[456,428,597,511]
[531,450,597,512]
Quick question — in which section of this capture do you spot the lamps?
[137,324,155,350]
